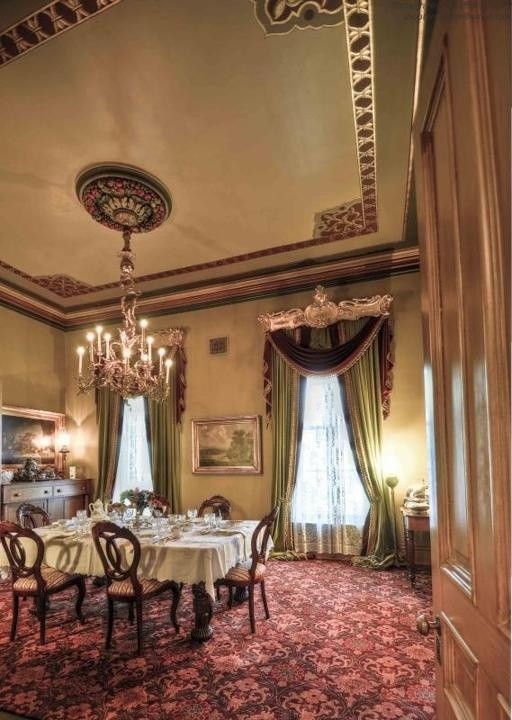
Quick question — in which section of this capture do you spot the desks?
[400,507,430,589]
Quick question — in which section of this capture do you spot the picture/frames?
[207,334,229,356]
[0,404,65,472]
[191,414,261,474]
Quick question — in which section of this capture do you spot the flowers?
[120,488,170,516]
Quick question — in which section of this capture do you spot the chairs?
[198,495,232,520]
[92,521,181,656]
[17,503,50,529]
[213,506,279,632]
[0,521,85,644]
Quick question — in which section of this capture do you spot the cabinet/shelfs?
[0,479,93,529]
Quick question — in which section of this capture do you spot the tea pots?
[89,499,110,521]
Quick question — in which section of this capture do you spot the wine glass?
[75,509,215,534]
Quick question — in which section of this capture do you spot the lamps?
[385,476,405,568]
[74,161,172,402]
[59,444,70,470]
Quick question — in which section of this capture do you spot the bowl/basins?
[0,469,16,484]
[405,502,429,513]
